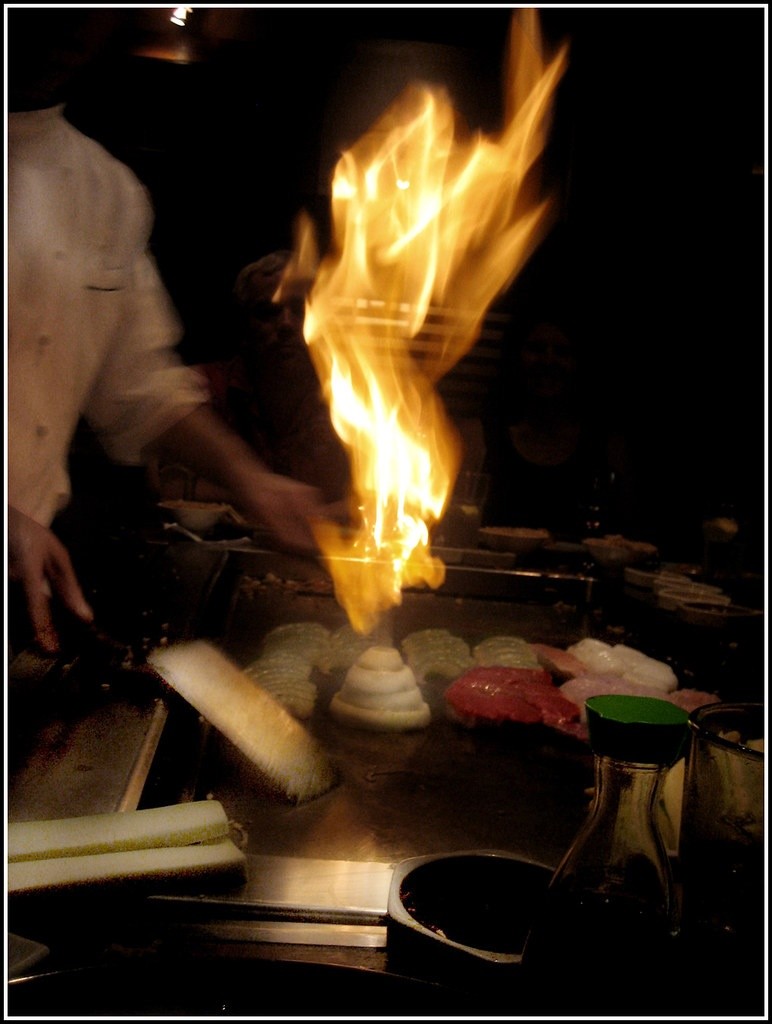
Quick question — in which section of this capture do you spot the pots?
[680,600,764,626]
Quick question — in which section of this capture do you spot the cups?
[677,702,764,934]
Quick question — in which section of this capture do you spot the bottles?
[520,694,688,1016]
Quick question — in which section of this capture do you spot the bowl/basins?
[477,527,550,555]
[582,538,657,566]
[157,500,230,529]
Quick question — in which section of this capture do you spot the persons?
[3,9,325,653]
[148,249,626,531]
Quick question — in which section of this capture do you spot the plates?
[430,545,516,568]
